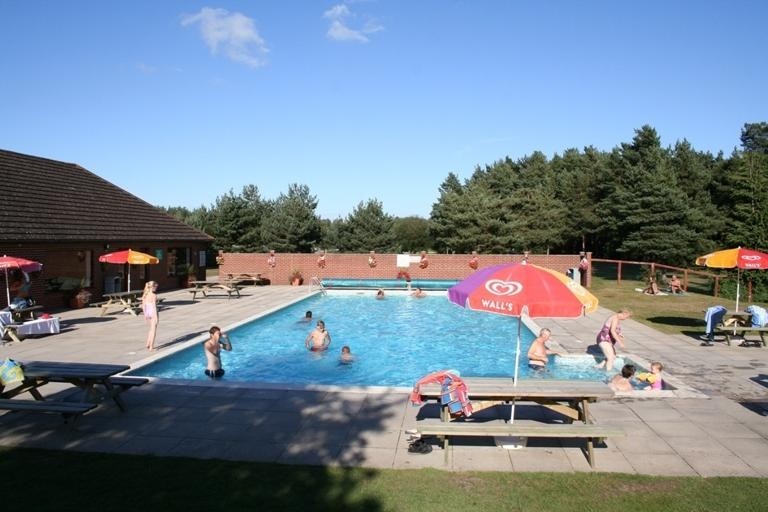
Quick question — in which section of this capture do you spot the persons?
[410,288,425,298]
[342,346,351,360]
[375,290,385,300]
[637,362,662,390]
[142,281,159,351]
[608,365,636,390]
[644,282,660,295]
[306,320,331,351]
[205,326,232,380]
[594,309,630,371]
[668,274,685,296]
[305,311,312,321]
[10,275,32,300]
[527,328,559,370]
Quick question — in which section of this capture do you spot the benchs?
[128,297,166,317]
[189,288,234,302]
[24,376,149,386]
[713,327,768,346]
[2,315,61,343]
[89,298,128,314]
[0,399,98,441]
[418,423,626,469]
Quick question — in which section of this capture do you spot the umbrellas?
[696,246,768,336]
[447,260,598,425]
[0,254,43,306]
[99,249,159,292]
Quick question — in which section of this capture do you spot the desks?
[100,290,143,317]
[702,309,768,337]
[3,361,130,414]
[189,281,242,299]
[0,304,49,321]
[418,377,615,424]
